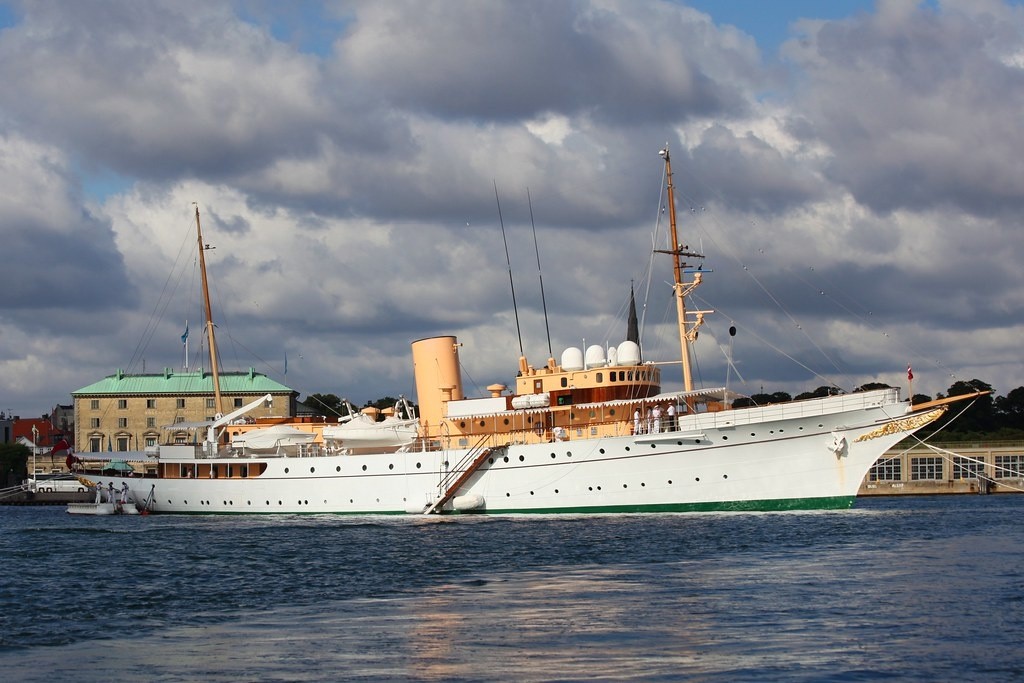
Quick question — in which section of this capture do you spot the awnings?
[443,386,751,422]
[160,421,216,431]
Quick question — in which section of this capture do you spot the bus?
[28,472,88,492]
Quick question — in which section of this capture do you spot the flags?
[907,362,913,380]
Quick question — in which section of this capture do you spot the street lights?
[32,425,39,492]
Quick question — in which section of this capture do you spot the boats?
[66,501,138,516]
[75,140,995,515]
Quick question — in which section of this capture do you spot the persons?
[187,468,193,479]
[94,481,102,504]
[209,467,216,479]
[632,402,675,436]
[107,482,117,504]
[121,482,129,504]
